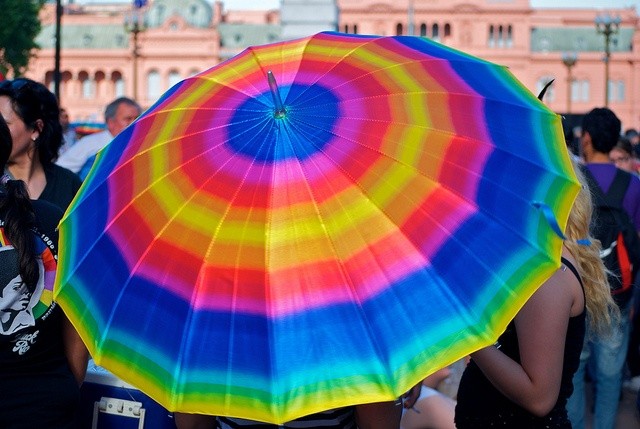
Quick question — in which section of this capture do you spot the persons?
[575,105,637,429]
[392,374,426,416]
[56,95,143,177]
[1,76,89,218]
[53,109,79,155]
[609,135,639,173]
[626,125,639,149]
[1,111,88,429]
[174,398,404,428]
[1,248,41,344]
[452,151,620,429]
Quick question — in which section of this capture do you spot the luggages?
[80,358,173,429]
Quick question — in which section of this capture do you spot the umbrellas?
[51,32,587,426]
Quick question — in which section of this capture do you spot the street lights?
[563,50,579,113]
[124,14,146,100]
[594,16,622,109]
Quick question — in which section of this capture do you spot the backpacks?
[579,165,640,309]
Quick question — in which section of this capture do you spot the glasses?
[1,78,30,92]
